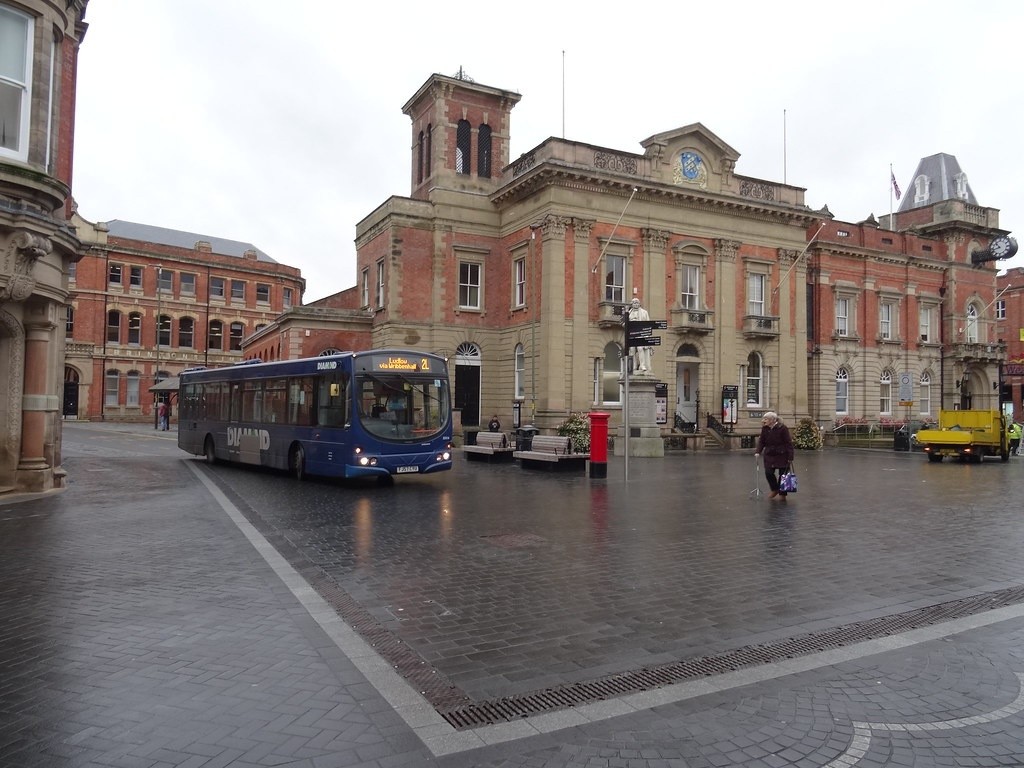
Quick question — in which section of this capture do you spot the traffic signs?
[628,335,661,347]
[628,328,652,336]
[628,320,667,329]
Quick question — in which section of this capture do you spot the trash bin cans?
[516,425,540,466]
[894,430,909,450]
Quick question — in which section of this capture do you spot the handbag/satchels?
[779,462,798,492]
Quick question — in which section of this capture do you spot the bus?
[176,347,455,490]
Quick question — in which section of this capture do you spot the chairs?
[371,403,387,418]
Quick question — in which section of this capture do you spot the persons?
[1008,420,1021,456]
[622,298,650,371]
[755,412,794,500]
[160,402,168,431]
[489,415,500,432]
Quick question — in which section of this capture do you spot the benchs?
[513,434,591,474]
[460,432,516,463]
[661,433,705,455]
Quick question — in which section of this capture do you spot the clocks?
[990,237,1011,258]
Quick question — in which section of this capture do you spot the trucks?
[913,405,1016,464]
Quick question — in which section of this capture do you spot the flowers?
[835,415,933,430]
[792,417,825,451]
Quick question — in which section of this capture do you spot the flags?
[892,174,902,200]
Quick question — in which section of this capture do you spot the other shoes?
[778,495,786,501]
[769,490,777,499]
[161,429,165,431]
[1012,453,1018,456]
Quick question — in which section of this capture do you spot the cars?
[893,421,932,452]
